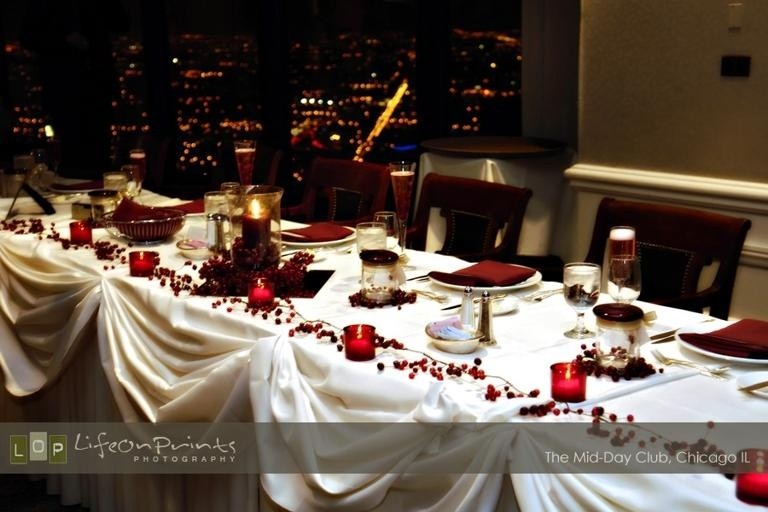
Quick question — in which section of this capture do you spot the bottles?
[477,289,494,345]
[591,302,645,372]
[88,188,119,229]
[210,214,232,262]
[359,249,400,304]
[459,284,475,330]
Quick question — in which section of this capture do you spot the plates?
[176,236,214,263]
[674,320,768,367]
[47,178,105,194]
[273,221,359,250]
[183,210,206,220]
[422,320,484,355]
[428,259,544,295]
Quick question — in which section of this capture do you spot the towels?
[679,319,768,359]
[160,199,204,213]
[52,180,104,190]
[271,223,354,242]
[429,259,536,286]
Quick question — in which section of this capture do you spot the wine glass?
[219,181,243,242]
[606,225,638,306]
[390,170,416,266]
[562,261,602,340]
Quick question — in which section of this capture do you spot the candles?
[248,277,275,308]
[70,222,92,244]
[130,251,153,277]
[550,363,586,403]
[343,325,375,361]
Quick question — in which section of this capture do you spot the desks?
[412,136,578,264]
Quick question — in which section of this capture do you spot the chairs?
[281,156,391,227]
[407,173,533,263]
[584,198,751,320]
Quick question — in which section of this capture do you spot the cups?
[341,323,377,362]
[101,146,147,203]
[69,219,94,246]
[607,253,642,308]
[128,250,159,278]
[245,275,276,308]
[234,137,257,185]
[547,359,587,406]
[354,221,388,255]
[370,209,400,256]
[202,190,232,252]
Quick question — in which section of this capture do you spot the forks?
[650,348,728,379]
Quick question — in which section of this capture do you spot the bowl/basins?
[99,206,187,247]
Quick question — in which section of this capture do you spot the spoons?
[667,356,731,375]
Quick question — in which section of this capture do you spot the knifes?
[648,318,717,344]
